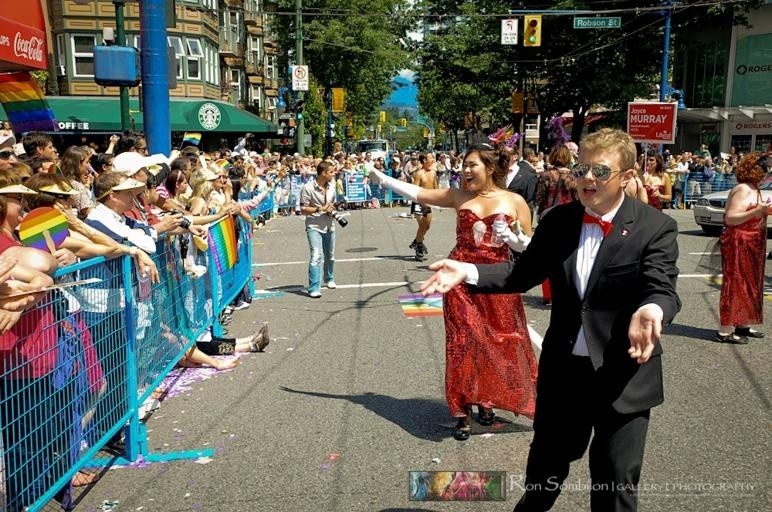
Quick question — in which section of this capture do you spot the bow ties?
[582,212,613,236]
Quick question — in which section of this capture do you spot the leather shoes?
[477,412,494,426]
[453,424,471,439]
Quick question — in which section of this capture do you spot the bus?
[356,139,388,160]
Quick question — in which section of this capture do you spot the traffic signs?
[572,16,621,29]
[416,115,427,125]
[524,15,542,48]
[500,17,518,46]
[292,64,310,92]
[626,103,677,145]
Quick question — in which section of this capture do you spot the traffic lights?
[293,97,304,122]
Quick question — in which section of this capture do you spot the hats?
[95,175,147,202]
[112,152,157,178]
[0,135,16,145]
[195,172,220,182]
[0,184,39,197]
[39,181,80,195]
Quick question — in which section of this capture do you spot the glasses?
[570,162,625,181]
[1,150,16,158]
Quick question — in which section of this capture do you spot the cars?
[692,176,772,237]
[391,149,417,159]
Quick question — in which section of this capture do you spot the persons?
[0,121,772,512]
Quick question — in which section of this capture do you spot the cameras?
[170,210,190,229]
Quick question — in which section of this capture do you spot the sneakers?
[714,331,748,344]
[734,325,764,338]
[410,239,428,261]
[327,281,337,289]
[310,291,322,298]
[250,324,270,351]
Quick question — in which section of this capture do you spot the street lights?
[666,85,689,112]
[275,85,298,123]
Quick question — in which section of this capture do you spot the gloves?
[362,160,423,203]
[494,220,531,252]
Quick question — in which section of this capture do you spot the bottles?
[328,207,348,228]
[491,208,508,248]
